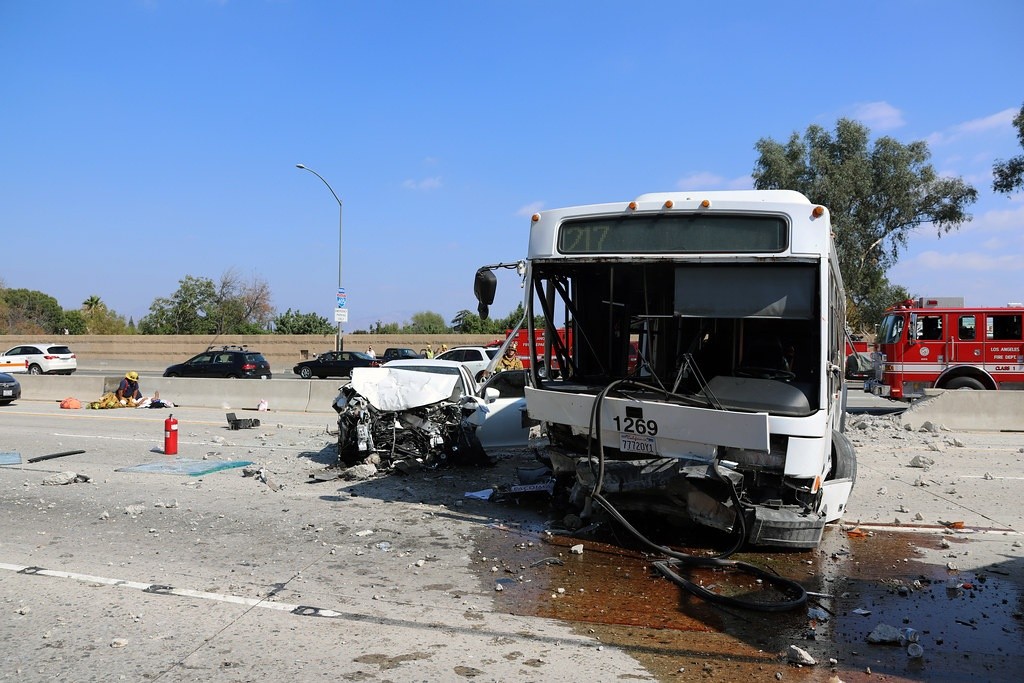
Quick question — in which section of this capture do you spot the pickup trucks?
[375,348,424,364]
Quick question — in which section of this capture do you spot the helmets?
[442,345,447,348]
[507,344,517,352]
[427,345,431,349]
[126,372,138,381]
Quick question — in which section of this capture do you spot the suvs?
[433,346,501,384]
[162,345,273,380]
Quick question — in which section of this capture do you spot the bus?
[473,191,858,550]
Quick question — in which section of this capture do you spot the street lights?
[296,164,344,359]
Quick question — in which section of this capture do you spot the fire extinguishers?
[165,414,178,455]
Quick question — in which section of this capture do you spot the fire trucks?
[863,296,1024,403]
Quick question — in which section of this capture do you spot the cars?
[0,372,22,406]
[293,352,386,379]
[331,359,531,473]
[0,343,77,375]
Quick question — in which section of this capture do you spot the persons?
[495,345,523,373]
[115,371,159,408]
[425,344,449,360]
[364,345,376,359]
